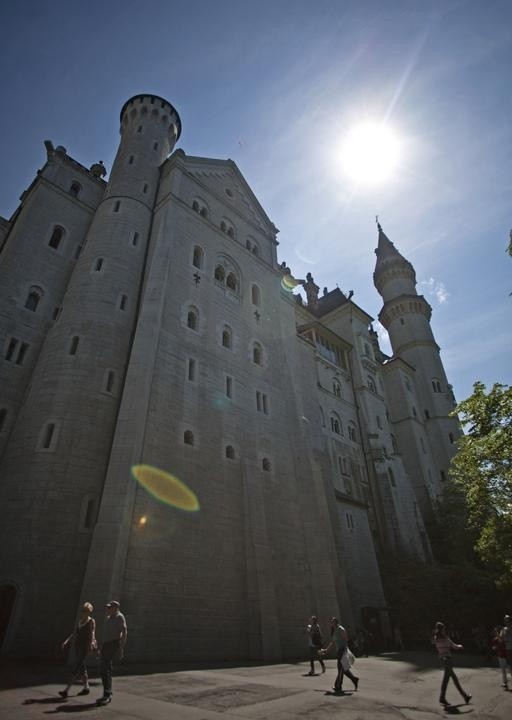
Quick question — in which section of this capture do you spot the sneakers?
[77,689,90,695]
[59,691,67,697]
[96,694,112,706]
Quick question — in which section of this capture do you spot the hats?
[106,600,120,607]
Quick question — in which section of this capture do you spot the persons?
[364,616,379,658]
[433,622,471,705]
[57,602,97,698]
[490,626,511,692]
[498,615,511,669]
[307,615,326,675]
[97,600,128,704]
[319,618,359,693]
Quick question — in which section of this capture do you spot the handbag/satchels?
[340,649,356,671]
